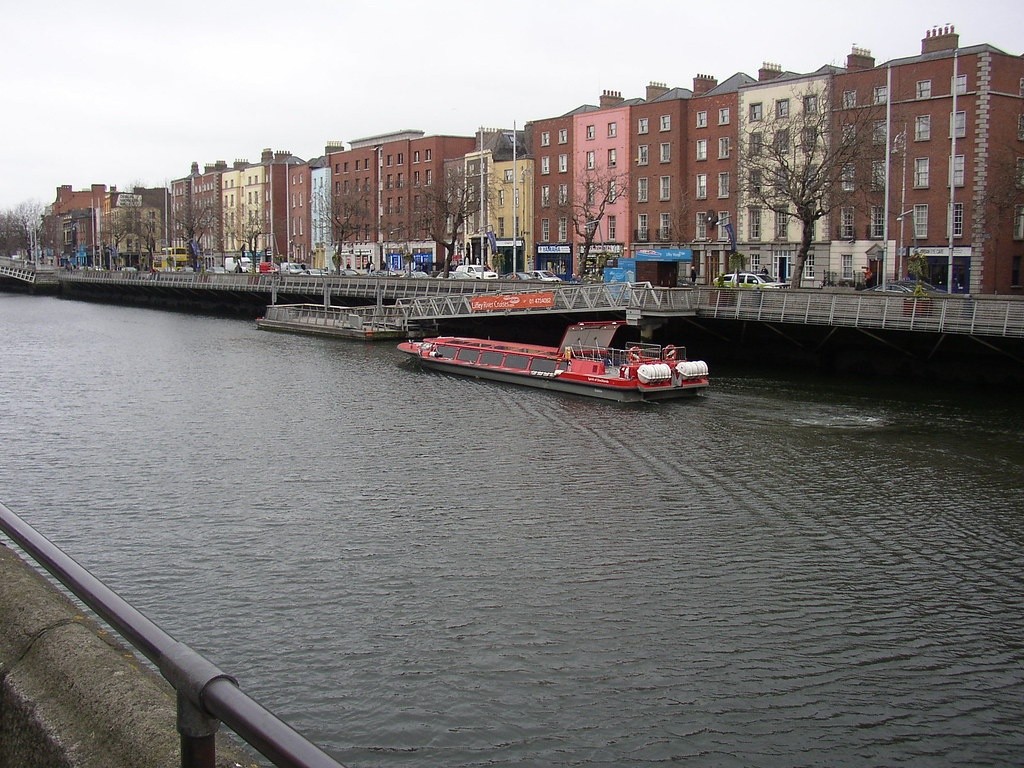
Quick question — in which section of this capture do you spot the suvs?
[281,262,308,276]
[713,274,792,290]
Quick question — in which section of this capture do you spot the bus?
[160,247,188,273]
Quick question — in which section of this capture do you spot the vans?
[225,257,252,273]
[456,265,499,280]
[260,262,280,274]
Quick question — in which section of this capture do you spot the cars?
[527,271,562,282]
[299,268,328,277]
[436,271,474,280]
[178,266,193,273]
[205,266,230,274]
[500,272,541,283]
[861,279,947,291]
[121,266,137,272]
[332,270,359,276]
[399,272,432,279]
[367,270,398,278]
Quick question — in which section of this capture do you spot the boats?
[397,321,710,404]
